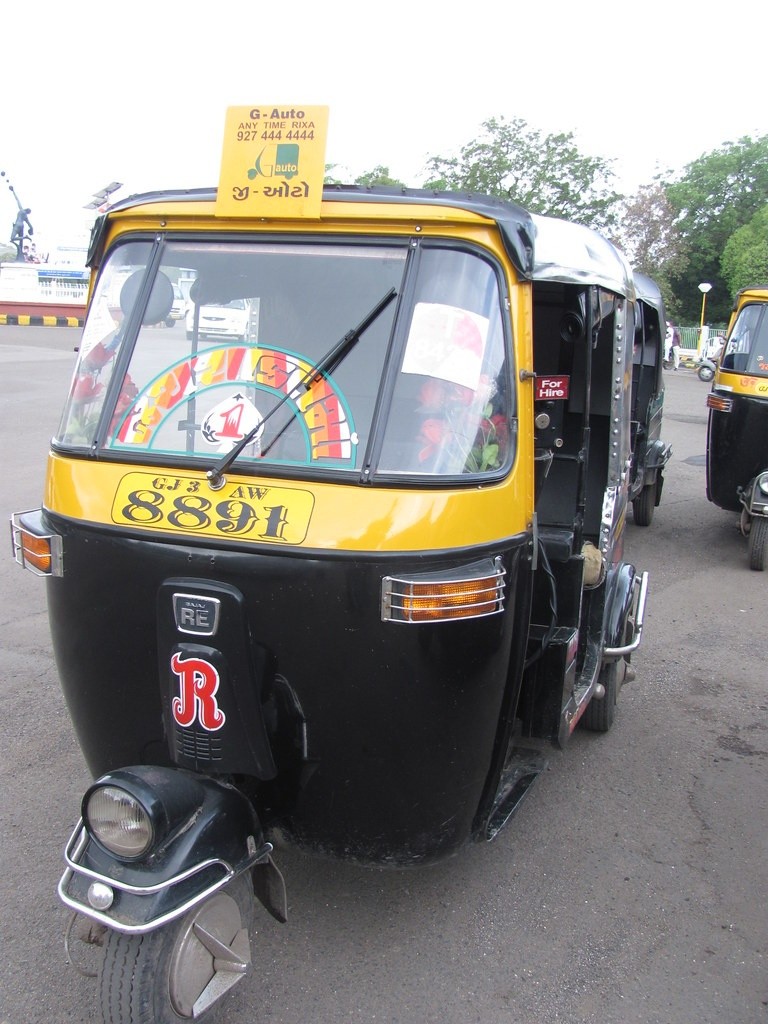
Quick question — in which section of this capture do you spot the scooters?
[662,348,676,370]
[698,342,728,382]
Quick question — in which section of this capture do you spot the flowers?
[412,375,509,473]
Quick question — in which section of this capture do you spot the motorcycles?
[8,183,653,1024]
[598,273,675,539]
[699,290,768,571]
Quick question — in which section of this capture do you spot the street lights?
[697,282,712,354]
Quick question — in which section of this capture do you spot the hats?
[666,321,670,326]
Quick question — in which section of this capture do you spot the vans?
[164,283,186,328]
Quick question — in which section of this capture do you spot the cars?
[185,295,251,344]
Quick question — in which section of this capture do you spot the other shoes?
[674,368,677,371]
[663,358,669,361]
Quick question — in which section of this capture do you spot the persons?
[662,321,681,371]
[9,186,33,248]
